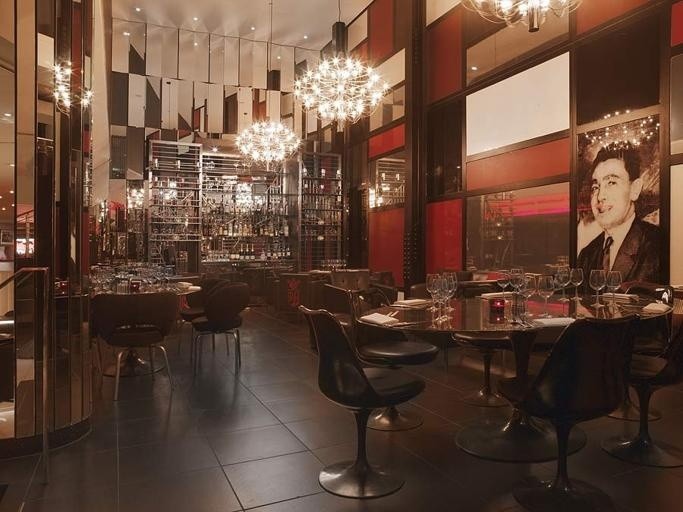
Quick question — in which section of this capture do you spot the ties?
[601,236,613,274]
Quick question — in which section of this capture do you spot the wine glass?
[424,254,623,324]
[317,259,348,271]
[88,259,175,293]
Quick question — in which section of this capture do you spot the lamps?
[237,1,300,165]
[459,0,585,34]
[289,1,391,124]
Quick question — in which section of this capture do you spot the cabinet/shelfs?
[200,153,300,272]
[302,153,345,271]
[147,137,197,276]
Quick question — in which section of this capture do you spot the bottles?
[148,159,342,260]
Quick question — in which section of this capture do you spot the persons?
[576,141,668,296]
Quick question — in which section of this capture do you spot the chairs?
[85,259,251,403]
[243,267,683,512]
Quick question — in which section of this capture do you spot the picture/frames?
[567,104,670,291]
